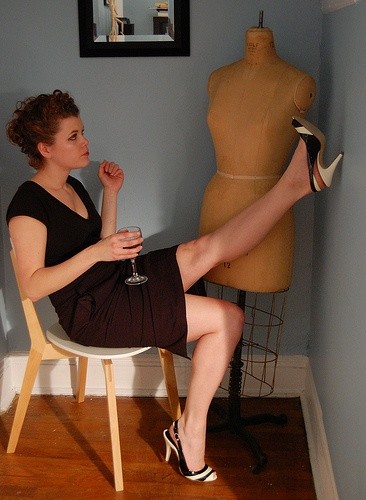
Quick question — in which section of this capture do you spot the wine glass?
[117,226,148,286]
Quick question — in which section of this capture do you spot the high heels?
[164,421,217,481]
[291,116,343,191]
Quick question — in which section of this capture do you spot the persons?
[200,27,316,293]
[4,89,345,482]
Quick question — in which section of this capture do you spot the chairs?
[6,237,183,491]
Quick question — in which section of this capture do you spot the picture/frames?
[78,0,190,57]
